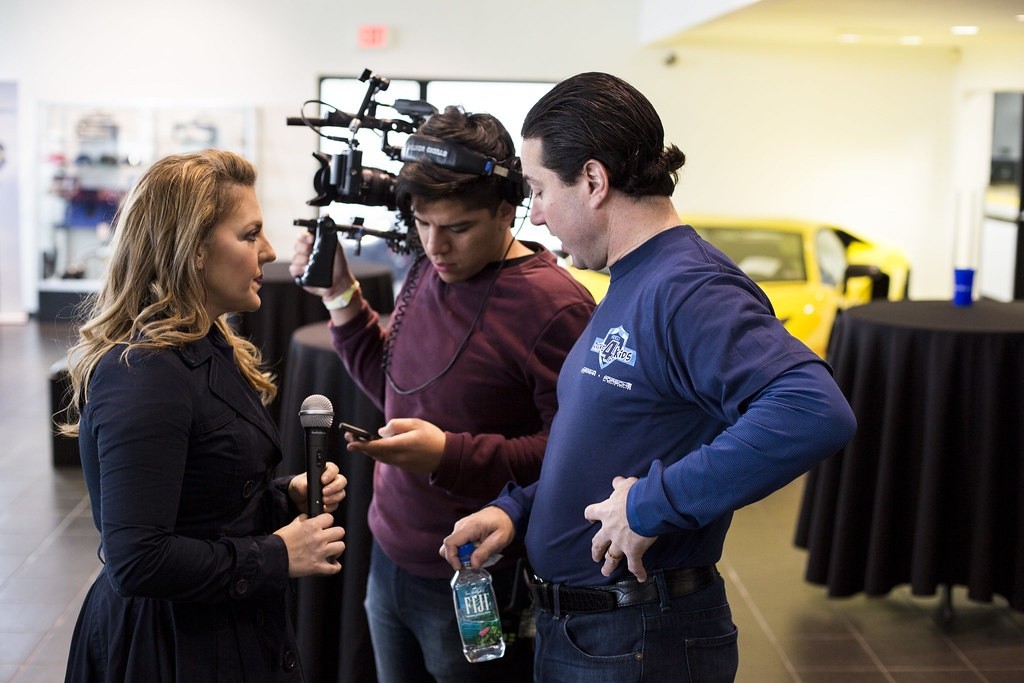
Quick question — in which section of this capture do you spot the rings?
[606,550,623,560]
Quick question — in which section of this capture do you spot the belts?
[524,563,720,612]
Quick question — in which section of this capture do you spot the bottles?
[450,543,505,664]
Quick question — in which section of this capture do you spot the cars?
[564,218,911,364]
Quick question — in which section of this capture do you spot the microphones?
[298,394,334,516]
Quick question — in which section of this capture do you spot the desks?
[792,300,1023,633]
[276,314,398,682]
[226,262,394,430]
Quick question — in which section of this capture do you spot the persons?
[293,112,597,683]
[438,72,858,683]
[64,148,349,683]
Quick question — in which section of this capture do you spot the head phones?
[402,134,532,206]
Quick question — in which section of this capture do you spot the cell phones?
[338,423,378,441]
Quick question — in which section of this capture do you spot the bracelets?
[322,280,360,310]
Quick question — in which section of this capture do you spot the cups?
[953,268,976,306]
